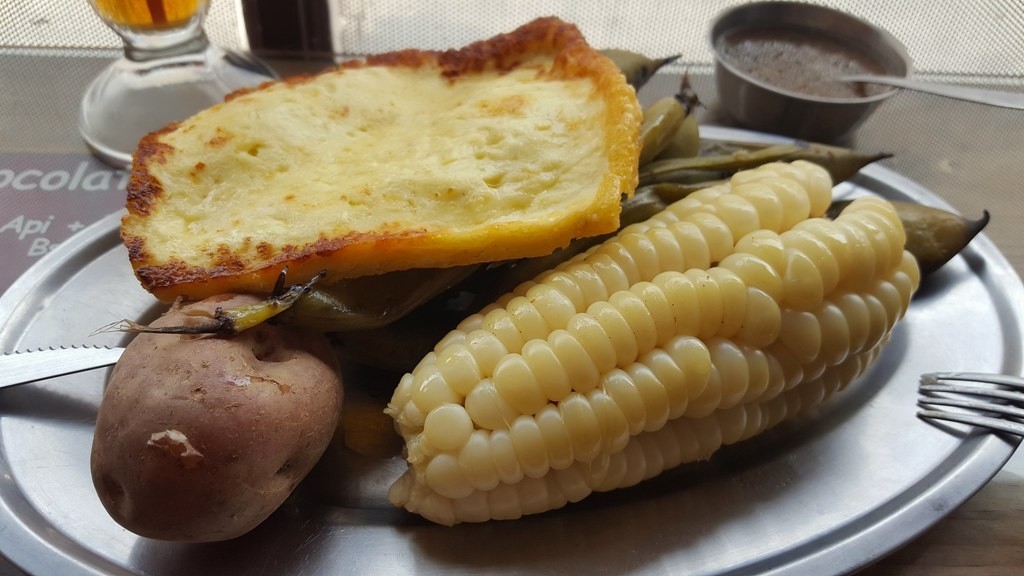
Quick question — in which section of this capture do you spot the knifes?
[0,345,127,390]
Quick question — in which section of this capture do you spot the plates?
[0,122,1024,574]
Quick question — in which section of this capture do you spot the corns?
[382,157,921,527]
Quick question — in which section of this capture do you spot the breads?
[117,15,643,305]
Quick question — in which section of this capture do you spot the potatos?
[90,293,344,544]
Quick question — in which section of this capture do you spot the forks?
[917,373,1024,440]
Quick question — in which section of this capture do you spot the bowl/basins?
[707,0,912,140]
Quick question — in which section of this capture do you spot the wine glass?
[77,0,287,162]
